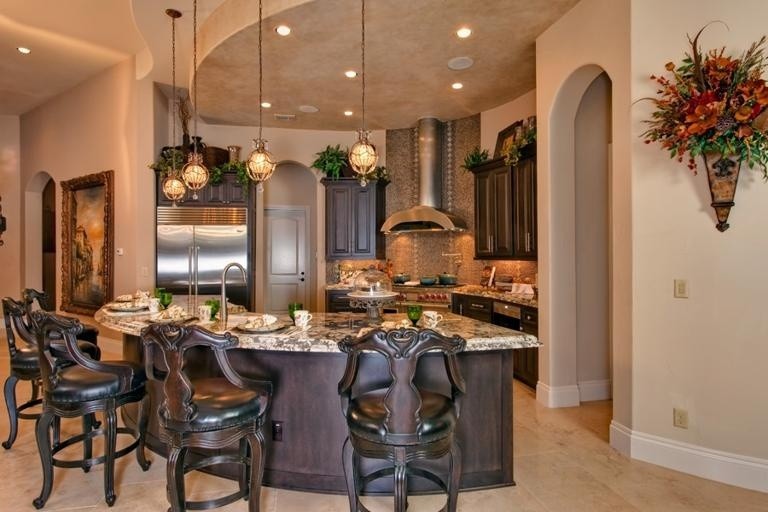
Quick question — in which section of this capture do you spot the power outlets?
[672,409,688,429]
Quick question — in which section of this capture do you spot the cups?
[423,311,442,329]
[197,305,211,323]
[293,310,312,326]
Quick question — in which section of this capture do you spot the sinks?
[227,312,256,326]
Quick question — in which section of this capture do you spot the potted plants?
[309,144,348,182]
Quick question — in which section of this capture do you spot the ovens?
[394,300,452,313]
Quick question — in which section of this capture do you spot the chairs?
[337,326,466,512]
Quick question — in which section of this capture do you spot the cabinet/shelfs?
[320,178,389,261]
[473,165,513,260]
[156,169,254,312]
[513,158,537,261]
[325,286,398,313]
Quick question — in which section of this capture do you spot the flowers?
[627,19,768,232]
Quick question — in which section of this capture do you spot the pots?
[420,272,457,285]
[394,272,410,283]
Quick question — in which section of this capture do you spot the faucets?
[221,263,248,318]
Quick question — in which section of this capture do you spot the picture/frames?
[492,120,520,159]
[60,169,115,318]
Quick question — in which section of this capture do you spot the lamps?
[180,0,210,201]
[245,0,276,192]
[348,0,378,188]
[161,8,187,209]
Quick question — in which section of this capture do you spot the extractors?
[381,118,467,234]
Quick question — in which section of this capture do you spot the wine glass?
[348,292,401,325]
[203,299,221,322]
[156,286,173,310]
[407,305,422,327]
[288,303,303,326]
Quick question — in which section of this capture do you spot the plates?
[237,320,286,332]
[109,295,193,324]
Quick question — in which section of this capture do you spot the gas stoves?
[392,281,466,288]
